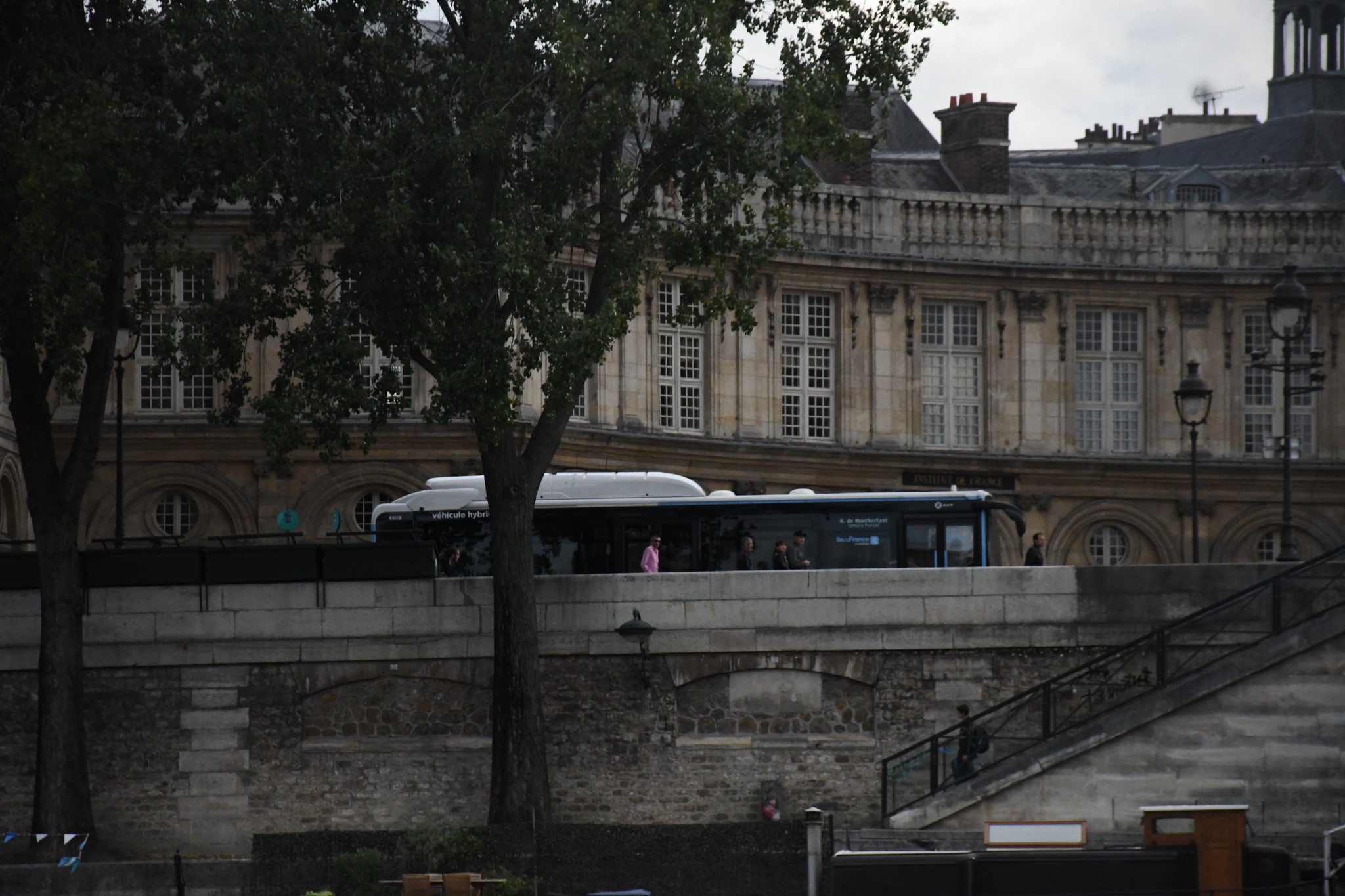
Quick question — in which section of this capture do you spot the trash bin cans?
[1140,805,1250,896]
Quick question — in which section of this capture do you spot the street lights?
[105,298,144,554]
[1264,261,1314,562]
[1173,360,1213,563]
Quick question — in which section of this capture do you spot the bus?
[371,469,1028,581]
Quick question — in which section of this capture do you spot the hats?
[956,703,970,716]
[793,527,807,537]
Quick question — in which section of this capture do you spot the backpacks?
[971,724,990,754]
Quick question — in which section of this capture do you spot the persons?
[443,545,461,577]
[772,531,811,570]
[640,535,661,572]
[738,537,754,571]
[1024,533,1044,566]
[957,704,977,780]
[763,797,779,820]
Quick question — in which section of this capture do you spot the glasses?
[650,539,661,542]
